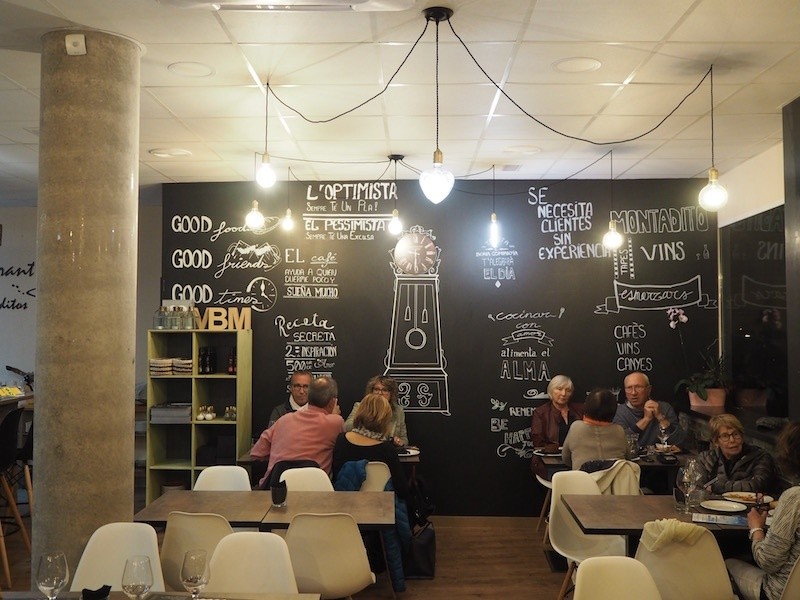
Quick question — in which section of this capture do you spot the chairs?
[191,465,253,491]
[343,459,401,583]
[69,521,167,594]
[201,531,299,595]
[285,511,377,600]
[534,472,552,545]
[547,468,629,600]
[573,555,661,600]
[162,509,234,594]
[279,468,336,492]
[260,459,321,491]
[633,519,735,600]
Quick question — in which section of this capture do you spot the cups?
[625,434,638,461]
[270,486,288,511]
[646,445,655,456]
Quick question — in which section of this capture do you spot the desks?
[536,442,770,558]
[133,487,397,537]
[399,444,421,463]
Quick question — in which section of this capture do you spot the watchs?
[748,527,764,540]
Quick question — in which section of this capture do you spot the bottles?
[198,343,217,374]
[226,357,237,375]
[196,405,216,420]
[224,406,237,421]
[152,306,194,330]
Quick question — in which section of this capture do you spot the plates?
[398,449,421,457]
[699,499,747,511]
[532,448,562,457]
[722,492,774,504]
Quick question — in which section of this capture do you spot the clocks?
[392,231,440,276]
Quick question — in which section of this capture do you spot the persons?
[532,372,800,600]
[250,370,409,498]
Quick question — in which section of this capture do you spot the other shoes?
[416,504,436,526]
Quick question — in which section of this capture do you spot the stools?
[0,403,32,588]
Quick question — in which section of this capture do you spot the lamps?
[419,5,457,206]
[255,81,278,190]
[488,165,500,250]
[696,63,731,214]
[244,152,266,231]
[602,152,624,253]
[281,166,297,231]
[388,158,402,236]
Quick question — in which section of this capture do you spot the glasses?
[373,387,390,395]
[717,430,742,442]
[293,384,308,391]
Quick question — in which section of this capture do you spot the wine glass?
[657,424,671,452]
[35,550,70,600]
[675,466,697,516]
[121,556,154,600]
[179,550,210,600]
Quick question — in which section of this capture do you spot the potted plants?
[669,368,730,408]
[734,369,784,409]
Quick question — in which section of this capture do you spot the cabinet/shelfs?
[142,325,255,508]
